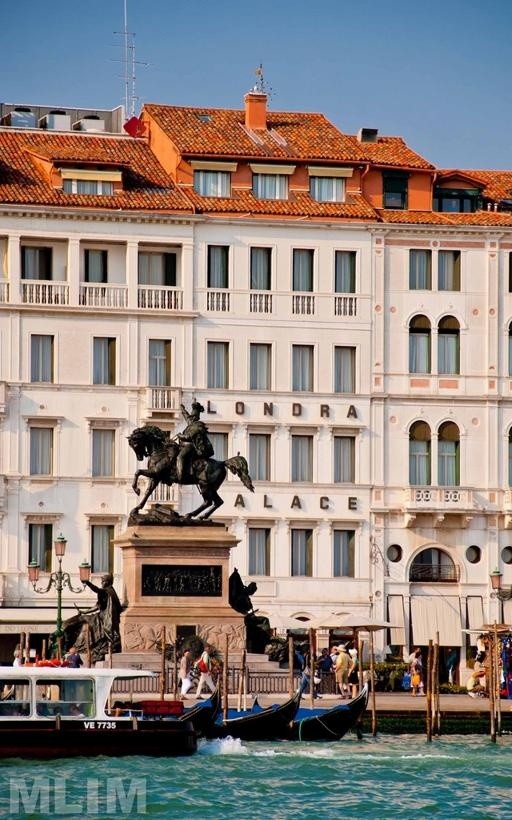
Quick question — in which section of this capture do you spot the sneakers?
[177,694,205,700]
[299,695,354,700]
[468,692,475,699]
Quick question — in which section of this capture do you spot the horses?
[125,426,255,521]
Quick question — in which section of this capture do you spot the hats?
[202,644,211,647]
[335,645,347,652]
[348,648,358,657]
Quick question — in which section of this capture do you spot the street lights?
[489,566,512,625]
[26,532,93,666]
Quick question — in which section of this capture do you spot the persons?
[178,648,193,700]
[12,703,85,717]
[60,574,122,664]
[297,635,489,700]
[194,646,216,700]
[169,402,207,481]
[13,649,22,667]
[63,647,83,668]
[244,582,257,596]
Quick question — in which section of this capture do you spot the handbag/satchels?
[303,655,310,676]
[199,654,210,673]
[412,672,420,686]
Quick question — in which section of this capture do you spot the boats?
[109,677,221,740]
[0,666,197,761]
[251,674,369,741]
[176,678,301,741]
